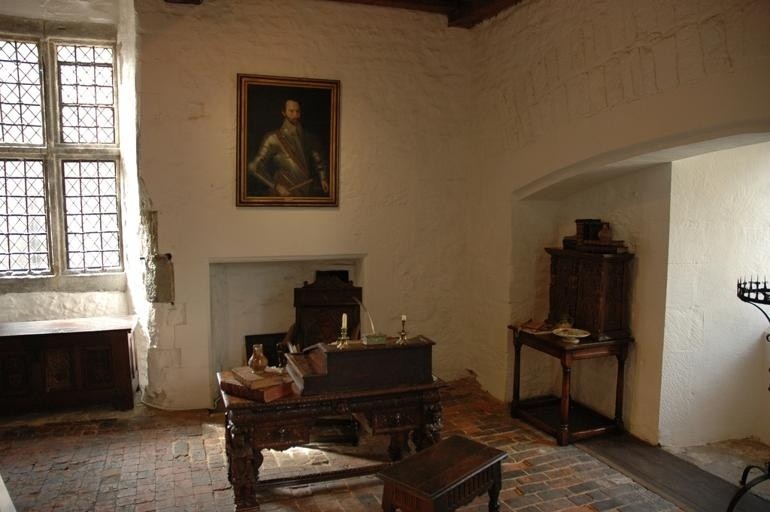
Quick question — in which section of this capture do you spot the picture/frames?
[233,71,339,208]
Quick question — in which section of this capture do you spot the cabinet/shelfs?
[542,242,635,334]
[2,319,142,418]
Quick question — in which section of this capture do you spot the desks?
[510,320,628,448]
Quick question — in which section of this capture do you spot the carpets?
[512,392,770,512]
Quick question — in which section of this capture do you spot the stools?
[376,435,508,512]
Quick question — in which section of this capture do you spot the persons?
[246,97,329,198]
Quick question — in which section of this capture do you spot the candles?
[341,312,348,329]
[401,314,407,320]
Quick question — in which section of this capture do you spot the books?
[219,370,296,405]
[231,365,283,390]
